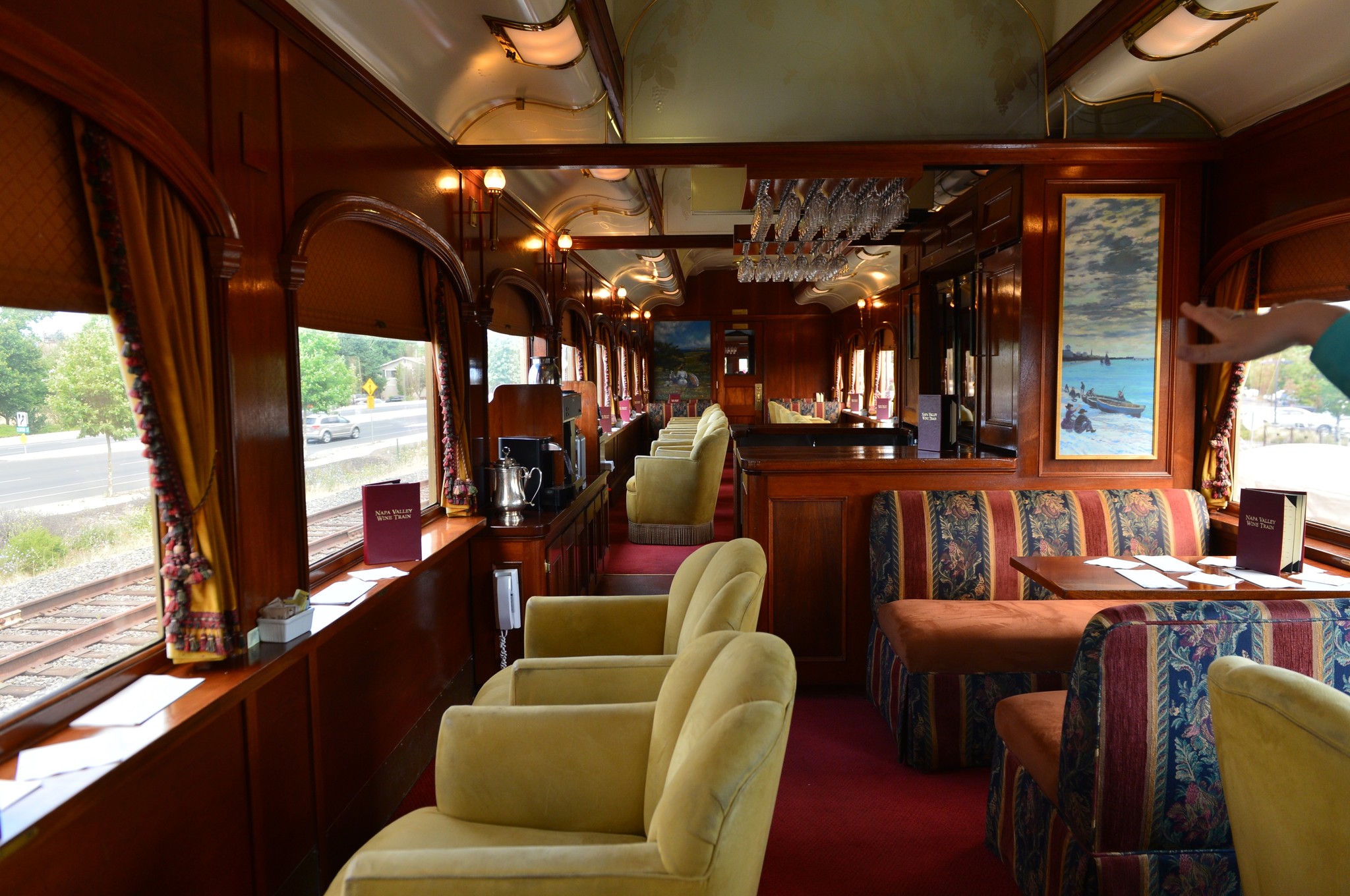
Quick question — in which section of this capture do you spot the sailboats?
[1100,353,1111,366]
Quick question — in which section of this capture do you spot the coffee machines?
[561,390,587,491]
[498,436,577,511]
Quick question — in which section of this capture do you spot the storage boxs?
[256,606,315,642]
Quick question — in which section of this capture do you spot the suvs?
[303,414,361,444]
[385,395,405,402]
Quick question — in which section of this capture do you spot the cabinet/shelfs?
[901,165,1018,459]
[494,468,609,661]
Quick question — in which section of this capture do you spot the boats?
[1082,394,1146,418]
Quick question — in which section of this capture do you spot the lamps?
[858,296,866,328]
[611,285,627,322]
[470,170,507,251]
[548,228,572,290]
[642,310,651,336]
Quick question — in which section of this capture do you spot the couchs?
[767,398,842,424]
[1203,652,1350,896]
[471,539,767,707]
[626,400,730,544]
[864,487,1212,766]
[981,599,1349,895]
[323,629,797,895]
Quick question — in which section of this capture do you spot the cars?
[1242,388,1350,438]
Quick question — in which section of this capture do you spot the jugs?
[489,446,542,522]
[528,356,564,390]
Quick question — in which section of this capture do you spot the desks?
[1009,554,1349,601]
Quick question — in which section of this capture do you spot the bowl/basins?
[256,607,315,644]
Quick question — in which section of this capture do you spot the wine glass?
[737,178,911,282]
